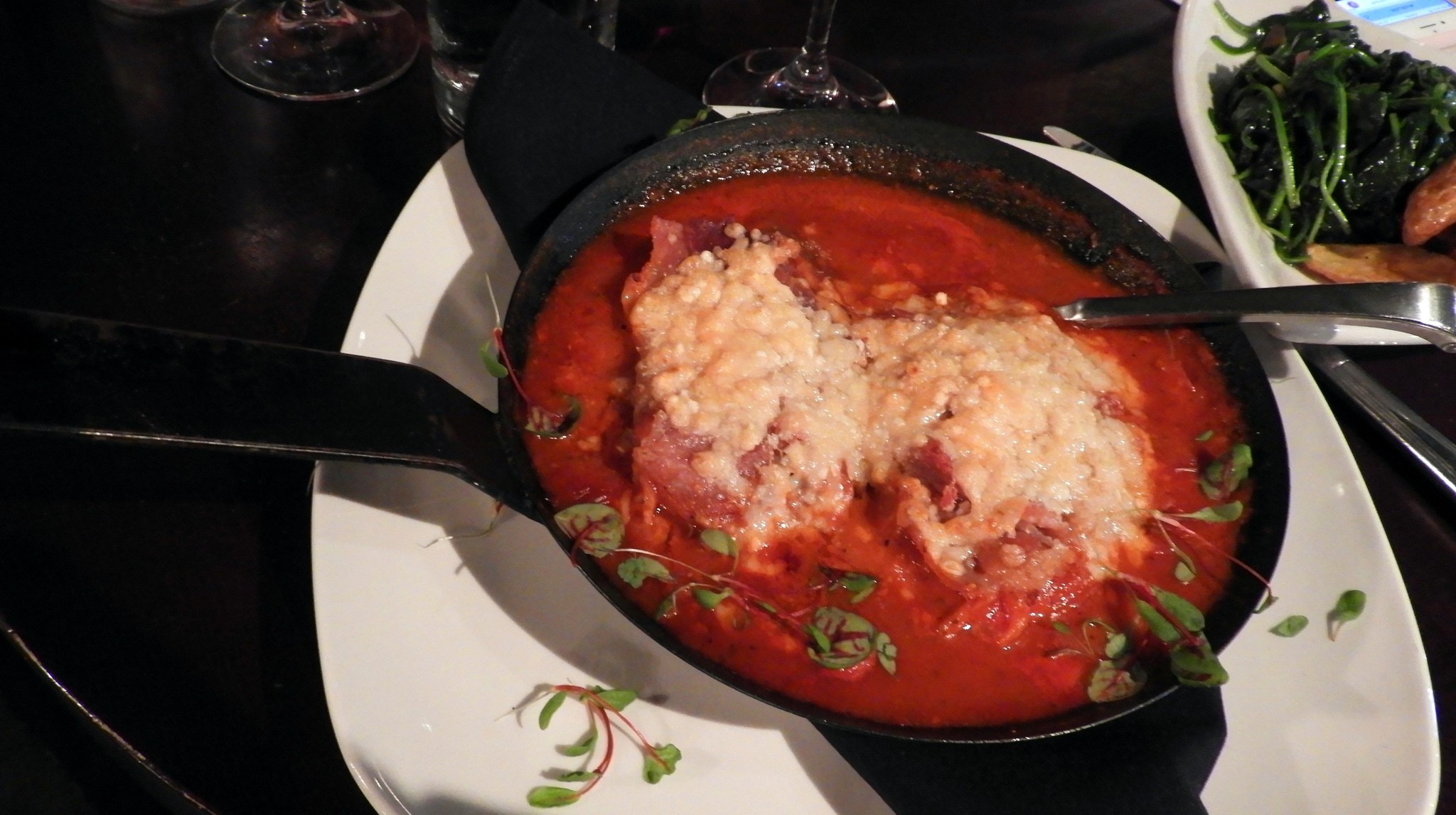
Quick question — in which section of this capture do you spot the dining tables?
[0,0,1455,815]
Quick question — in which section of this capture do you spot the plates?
[306,104,1443,815]
[1169,0,1456,346]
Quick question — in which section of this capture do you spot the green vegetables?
[1209,0,1456,263]
[484,326,1367,808]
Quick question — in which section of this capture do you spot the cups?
[423,0,621,142]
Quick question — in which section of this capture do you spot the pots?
[0,112,1293,746]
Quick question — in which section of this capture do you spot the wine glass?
[701,0,899,120]
[210,0,423,103]
